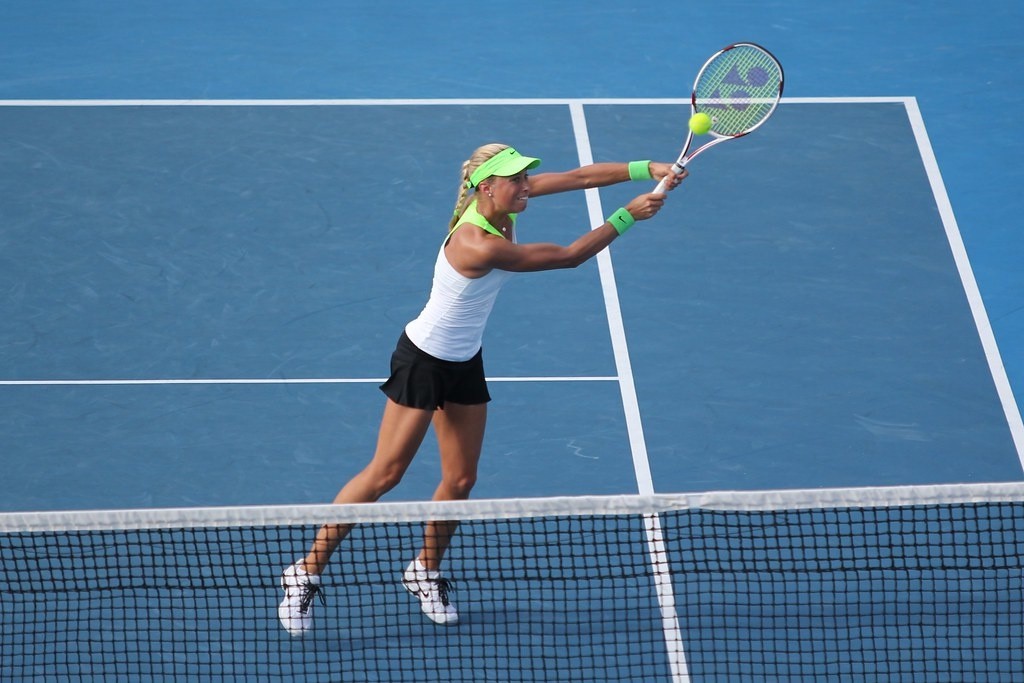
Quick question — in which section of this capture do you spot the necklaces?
[484,211,510,232]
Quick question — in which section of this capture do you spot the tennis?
[688,112,712,137]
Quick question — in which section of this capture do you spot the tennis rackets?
[653,40,786,195]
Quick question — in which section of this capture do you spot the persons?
[276,143,691,639]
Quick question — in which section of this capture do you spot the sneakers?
[401,561,459,624]
[278,557,327,637]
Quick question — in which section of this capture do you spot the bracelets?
[608,206,635,236]
[628,160,654,182]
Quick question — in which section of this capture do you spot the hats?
[469,147,540,187]
